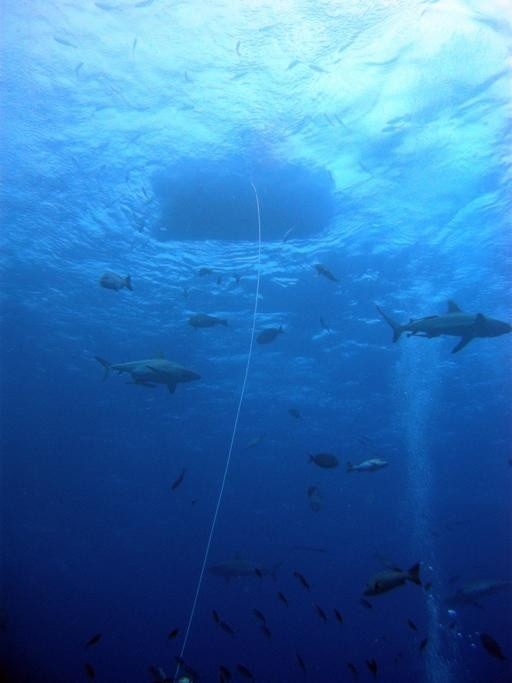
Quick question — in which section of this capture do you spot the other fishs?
[78,543,512,682]
[45,22,433,301]
